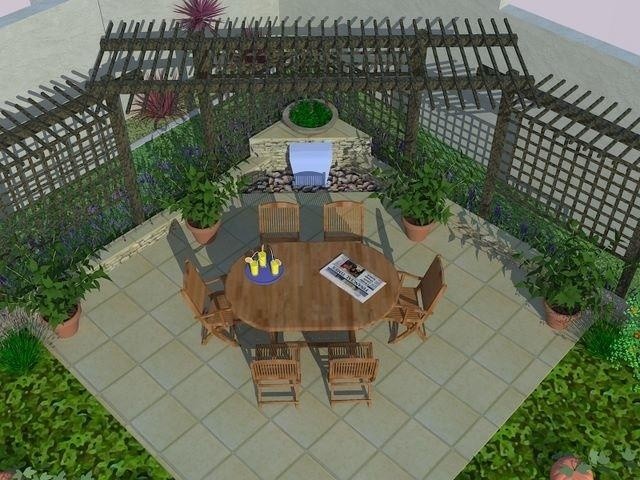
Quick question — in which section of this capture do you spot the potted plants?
[510,220,625,331]
[157,156,252,246]
[1,243,114,338]
[282,97,339,134]
[368,148,476,242]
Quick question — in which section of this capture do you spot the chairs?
[250,343,301,409]
[322,201,364,242]
[328,342,379,407]
[181,257,243,348]
[258,202,300,242]
[384,254,447,344]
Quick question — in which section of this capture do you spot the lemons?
[275,258,281,266]
[244,257,253,264]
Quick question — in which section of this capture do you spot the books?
[319,253,387,303]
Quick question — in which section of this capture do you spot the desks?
[225,240,402,343]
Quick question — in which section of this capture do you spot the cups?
[250,251,267,276]
[270,260,279,275]
